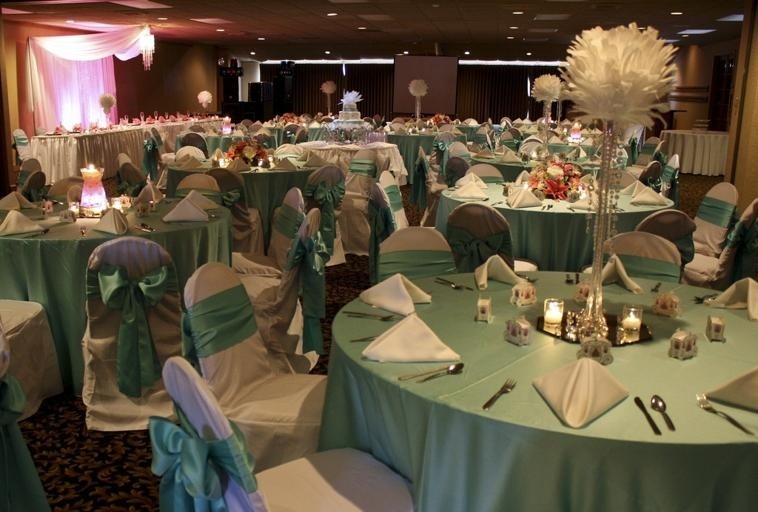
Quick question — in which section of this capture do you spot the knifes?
[342,310,384,318]
[576,273,581,286]
[481,181,639,214]
[23,197,219,240]
[399,365,450,380]
[634,396,663,437]
[437,277,473,290]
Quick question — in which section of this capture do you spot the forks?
[482,376,518,411]
[695,392,754,435]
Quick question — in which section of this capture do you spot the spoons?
[345,313,396,322]
[650,393,677,432]
[416,362,466,385]
[651,281,662,292]
[694,292,719,300]
[434,279,464,290]
[565,274,574,283]
[516,272,539,284]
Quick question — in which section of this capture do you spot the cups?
[618,302,644,331]
[542,297,565,324]
[566,307,582,334]
[542,324,561,337]
[520,152,530,166]
[616,327,641,344]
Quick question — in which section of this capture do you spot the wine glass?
[124,109,207,128]
